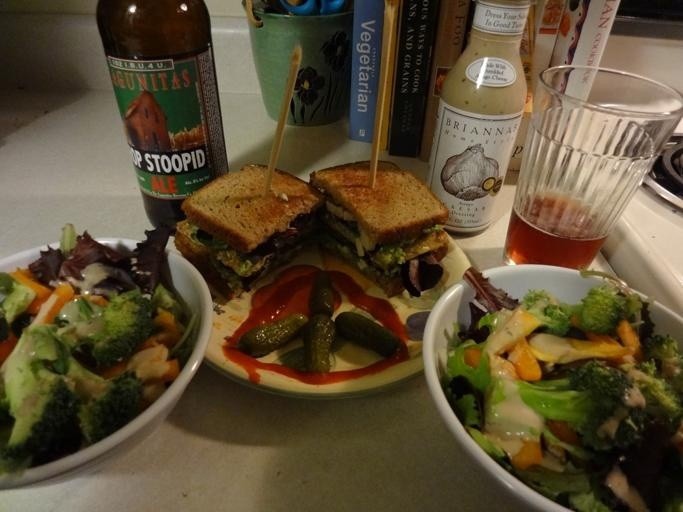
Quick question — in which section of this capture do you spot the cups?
[502,64,683,265]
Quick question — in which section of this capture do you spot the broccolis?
[0,271,197,473]
[442,283,682,512]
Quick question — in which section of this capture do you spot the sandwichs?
[174,160,449,298]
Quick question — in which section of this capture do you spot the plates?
[421,266,682,512]
[169,226,472,402]
[2,238,215,489]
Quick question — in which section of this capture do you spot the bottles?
[94,0,230,230]
[424,0,527,238]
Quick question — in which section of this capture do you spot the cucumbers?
[238,269,400,372]
